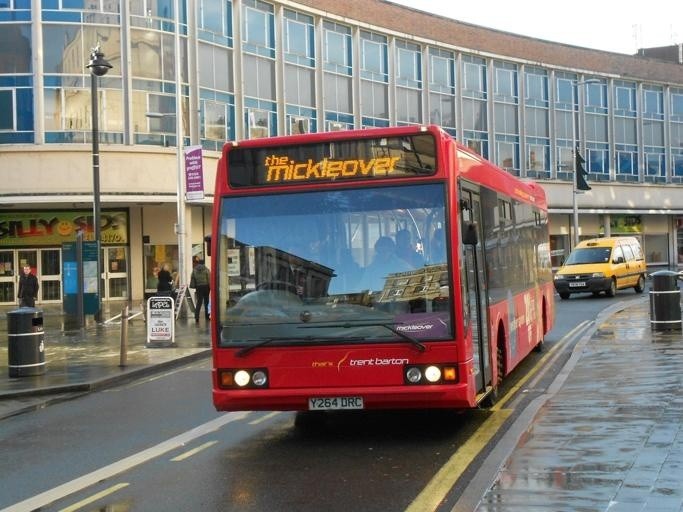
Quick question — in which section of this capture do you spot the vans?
[554,235,648,300]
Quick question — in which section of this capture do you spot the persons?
[258,246,325,298]
[17,263,39,307]
[156,262,172,296]
[190,259,210,322]
[395,230,428,312]
[339,237,418,316]
[430,228,448,266]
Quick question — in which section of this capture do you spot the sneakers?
[195,313,210,322]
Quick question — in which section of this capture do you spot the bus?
[211,123,554,415]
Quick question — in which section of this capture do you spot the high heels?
[188,277,197,288]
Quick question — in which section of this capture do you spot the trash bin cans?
[648,270,683,330]
[6,307,47,377]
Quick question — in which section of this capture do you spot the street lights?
[84,38,114,241]
